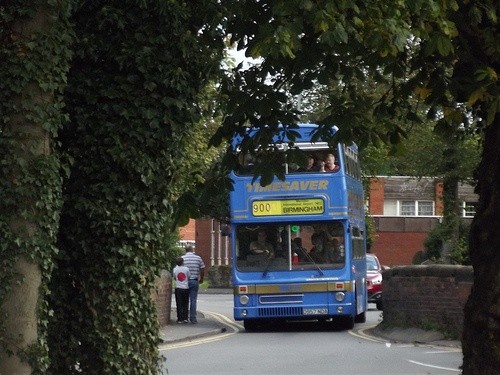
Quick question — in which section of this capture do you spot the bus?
[228,123,369,332]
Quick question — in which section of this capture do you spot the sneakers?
[178,321,182,324]
[192,320,198,324]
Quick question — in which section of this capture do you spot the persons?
[175,244,205,324]
[246,153,339,173]
[240,226,365,264]
[173,257,191,324]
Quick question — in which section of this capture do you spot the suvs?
[365,253,386,311]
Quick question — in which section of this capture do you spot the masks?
[184,320,188,323]
